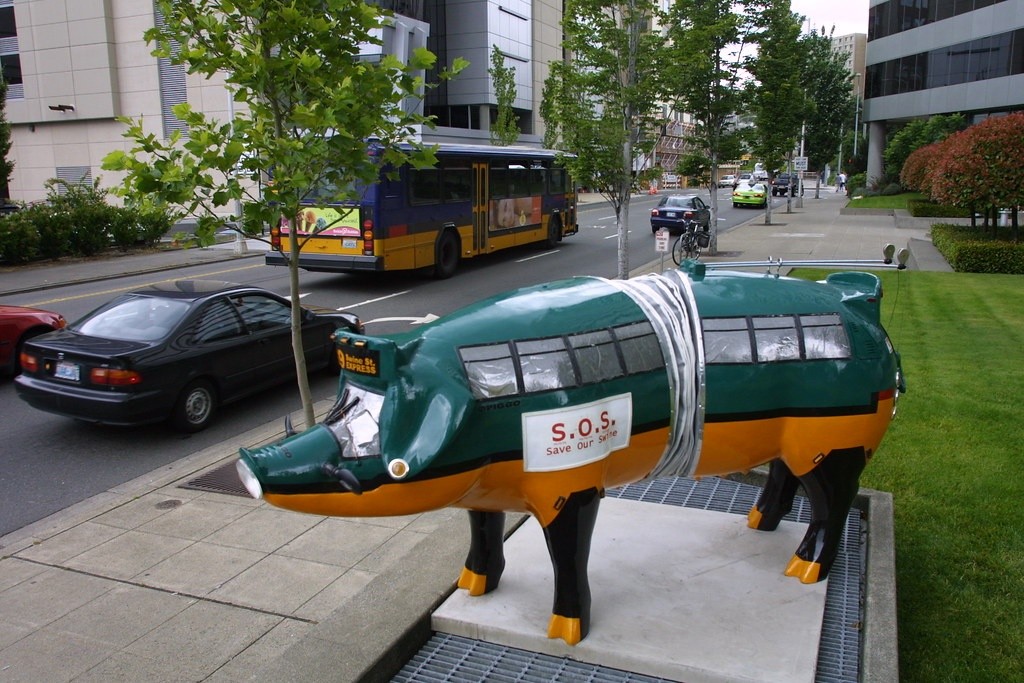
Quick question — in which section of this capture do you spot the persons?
[492,198,534,228]
[839,172,847,189]
[834,174,843,192]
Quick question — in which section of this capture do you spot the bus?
[754,162,780,181]
[265,135,582,280]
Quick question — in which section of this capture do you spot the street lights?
[854,72,860,157]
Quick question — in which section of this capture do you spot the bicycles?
[672,218,711,266]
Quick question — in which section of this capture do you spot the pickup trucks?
[771,172,804,197]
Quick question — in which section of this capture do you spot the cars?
[0,305,68,379]
[734,173,758,189]
[718,174,737,188]
[732,182,769,209]
[13,277,367,435]
[650,193,711,235]
